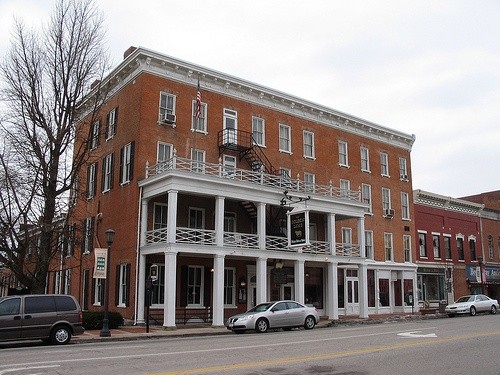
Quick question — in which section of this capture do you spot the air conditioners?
[400,174,408,179]
[160,113,176,124]
[386,209,395,216]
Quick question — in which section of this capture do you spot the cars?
[225,300,321,333]
[445,294,500,317]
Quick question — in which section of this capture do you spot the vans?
[0,294,85,346]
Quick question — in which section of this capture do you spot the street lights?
[477,255,484,294]
[99,225,115,336]
